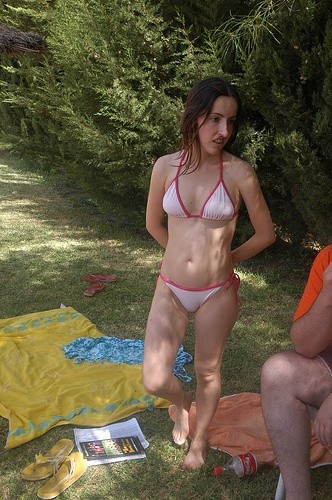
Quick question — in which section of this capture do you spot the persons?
[141,77,275,472]
[259,241,332,500]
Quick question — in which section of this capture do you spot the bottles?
[214,449,275,480]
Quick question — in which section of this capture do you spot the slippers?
[83,282,106,297]
[84,273,117,282]
[21,439,74,480]
[37,451,89,500]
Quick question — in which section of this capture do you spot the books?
[77,435,145,460]
